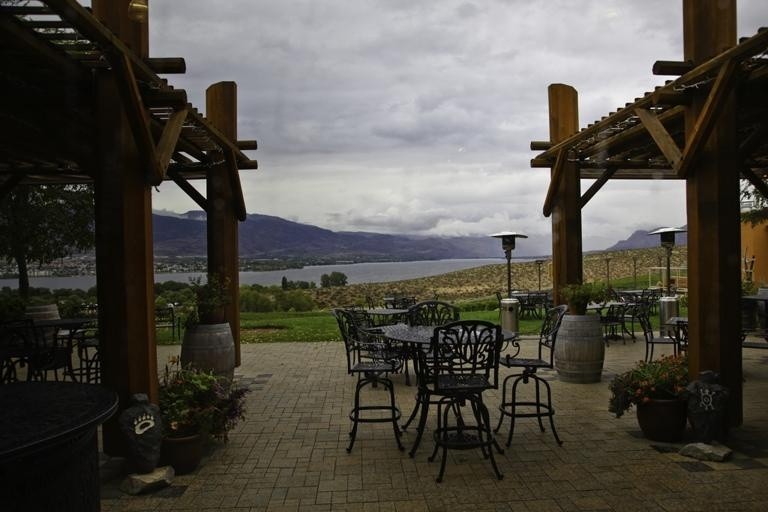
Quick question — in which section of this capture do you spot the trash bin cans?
[658,296,680,338]
[500,298,520,338]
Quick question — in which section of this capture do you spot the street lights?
[533,259,547,291]
[648,227,688,296]
[489,231,529,298]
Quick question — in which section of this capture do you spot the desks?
[510,293,546,320]
[587,300,637,347]
[618,290,650,302]
[355,308,409,327]
[662,316,688,327]
[384,324,517,458]
[8,317,91,382]
[0,378,121,512]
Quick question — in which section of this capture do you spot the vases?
[164,432,205,475]
[636,398,689,442]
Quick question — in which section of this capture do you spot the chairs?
[407,300,465,407]
[427,319,504,483]
[601,303,629,347]
[333,308,405,452]
[495,292,503,319]
[675,320,688,356]
[493,303,569,448]
[0,318,40,380]
[154,307,181,342]
[636,311,679,363]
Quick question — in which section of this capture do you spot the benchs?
[25,304,85,345]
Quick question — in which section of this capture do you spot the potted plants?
[559,282,607,315]
[187,269,231,323]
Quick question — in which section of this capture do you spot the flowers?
[607,353,689,418]
[159,354,252,444]
